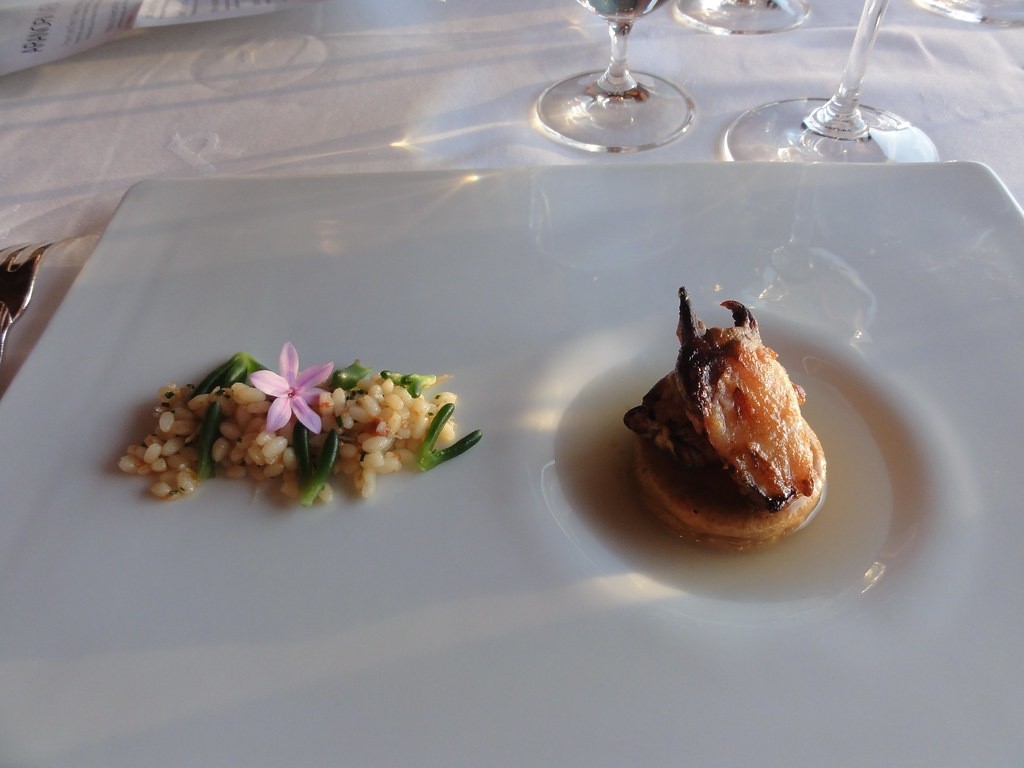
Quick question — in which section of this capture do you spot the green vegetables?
[191,350,486,509]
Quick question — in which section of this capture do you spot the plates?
[0,160,1024,766]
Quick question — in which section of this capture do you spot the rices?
[120,375,457,503]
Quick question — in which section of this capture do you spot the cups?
[908,0,1023,28]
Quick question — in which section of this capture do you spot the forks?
[0,232,101,368]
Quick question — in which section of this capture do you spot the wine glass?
[530,0,699,156]
[724,0,941,162]
[669,0,813,37]
